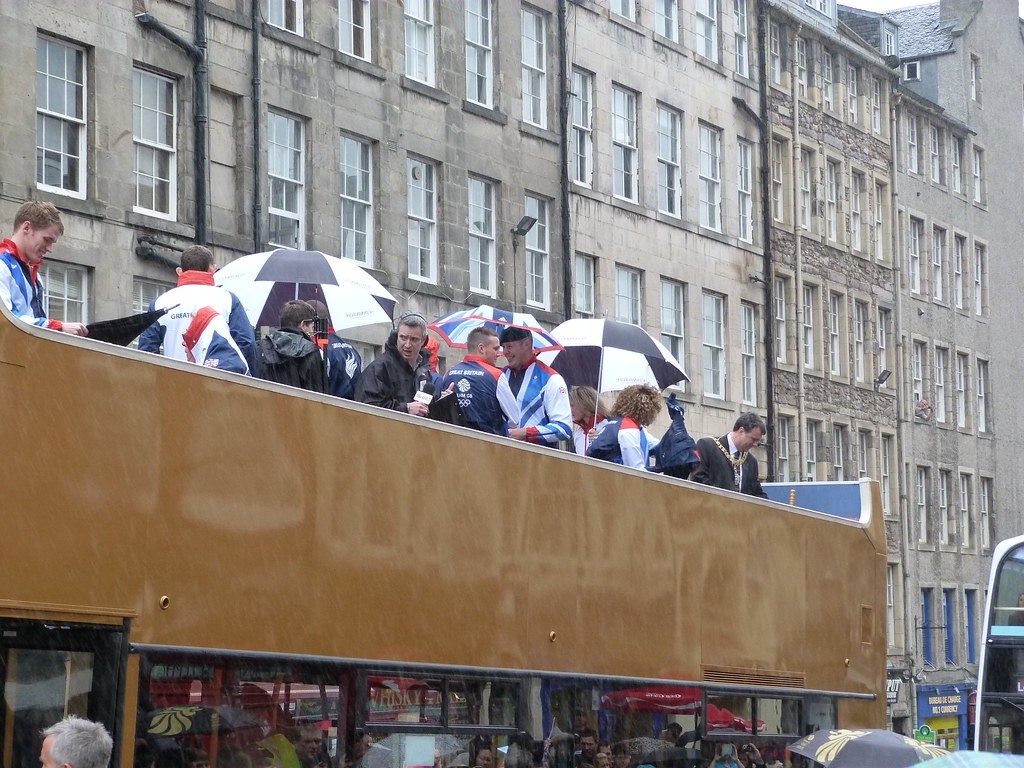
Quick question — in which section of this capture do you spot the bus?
[968,536,1024,756]
[0,304,887,768]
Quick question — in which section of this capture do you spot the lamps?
[874,369,892,392]
[947,685,959,694]
[929,686,940,696]
[510,216,538,236]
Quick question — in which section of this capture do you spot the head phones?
[389,312,428,348]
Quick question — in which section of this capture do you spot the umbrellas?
[428,304,565,353]
[214,244,403,334]
[786,726,1024,768]
[550,317,692,431]
[78,303,183,346]
[146,701,237,757]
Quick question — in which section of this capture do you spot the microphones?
[413,383,436,405]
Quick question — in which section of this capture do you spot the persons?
[249,300,361,400]
[139,245,263,376]
[692,411,771,499]
[574,722,691,768]
[914,399,933,422]
[569,384,663,469]
[354,312,455,417]
[1007,591,1024,625]
[441,328,575,443]
[38,714,113,768]
[708,741,765,768]
[295,724,331,768]
[475,746,493,768]
[0,200,89,335]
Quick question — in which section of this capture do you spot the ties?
[733,450,740,476]
